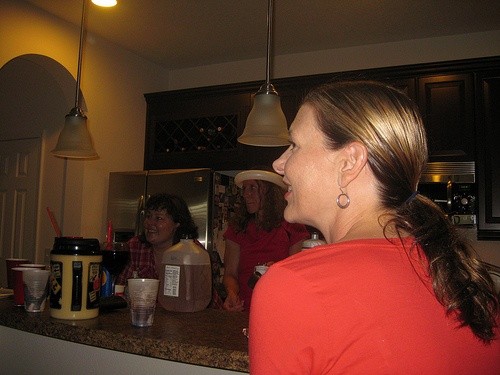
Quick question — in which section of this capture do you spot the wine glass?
[101,240,131,311]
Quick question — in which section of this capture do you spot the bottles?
[156,239,212,313]
[300,232,327,250]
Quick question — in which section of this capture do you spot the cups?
[254,265,268,276]
[113,281,125,298]
[16,263,47,270]
[127,278,160,328]
[40,243,54,264]
[11,267,41,307]
[22,270,50,312]
[5,259,30,290]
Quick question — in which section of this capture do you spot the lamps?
[51,0,99,160]
[238,0,291,147]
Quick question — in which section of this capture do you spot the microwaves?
[417,161,476,229]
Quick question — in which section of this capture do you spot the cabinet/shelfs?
[250,73,336,172]
[475,55,500,242]
[336,57,480,165]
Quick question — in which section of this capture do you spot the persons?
[247,83,500,375]
[111,193,220,311]
[223,159,310,312]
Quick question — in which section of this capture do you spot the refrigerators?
[106,168,248,312]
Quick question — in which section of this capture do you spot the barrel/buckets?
[49,237,103,320]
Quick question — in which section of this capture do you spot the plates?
[0,288,14,298]
